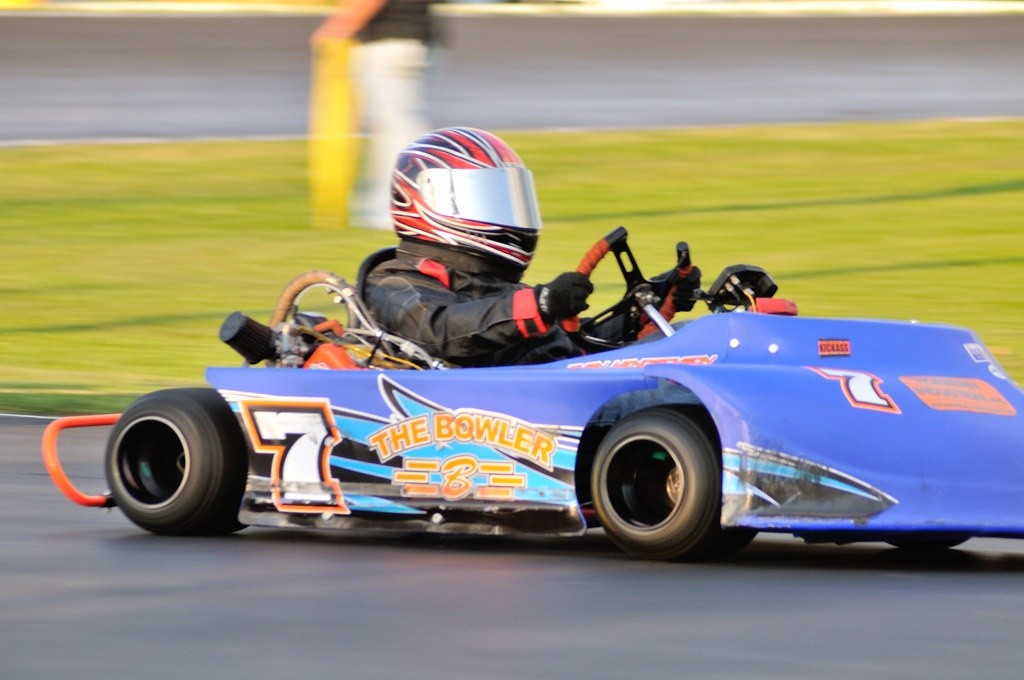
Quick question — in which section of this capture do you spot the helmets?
[389,126,540,281]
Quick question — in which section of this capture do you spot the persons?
[365,126,702,366]
[354,0,437,228]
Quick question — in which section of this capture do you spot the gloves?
[649,267,700,312]
[535,272,594,324]
